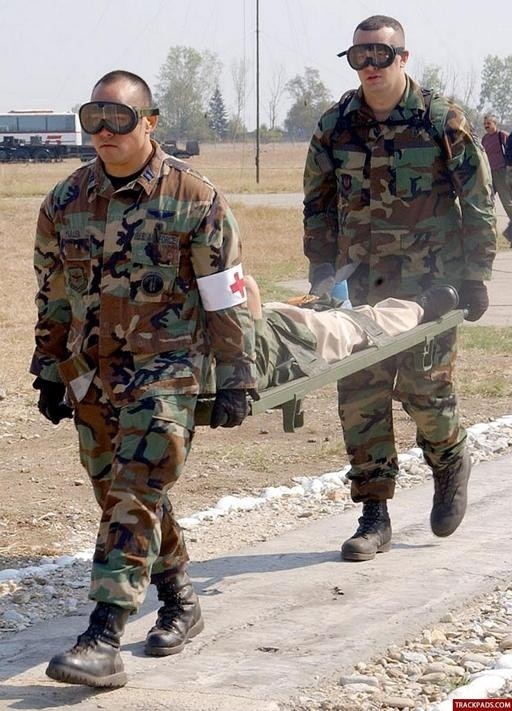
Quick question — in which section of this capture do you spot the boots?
[46,601,131,687]
[144,570,204,655]
[431,451,471,537]
[342,499,391,560]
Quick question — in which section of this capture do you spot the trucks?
[0,140,200,163]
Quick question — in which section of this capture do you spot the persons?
[302,15,498,561]
[30,70,259,691]
[502,131,512,247]
[200,262,461,396]
[482,116,511,221]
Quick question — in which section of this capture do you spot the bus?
[0,109,82,157]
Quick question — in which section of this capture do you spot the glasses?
[79,102,159,135]
[347,44,404,70]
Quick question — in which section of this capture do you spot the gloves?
[38,380,73,424]
[457,281,488,321]
[210,389,246,428]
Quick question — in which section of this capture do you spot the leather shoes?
[309,263,334,295]
[413,284,460,322]
